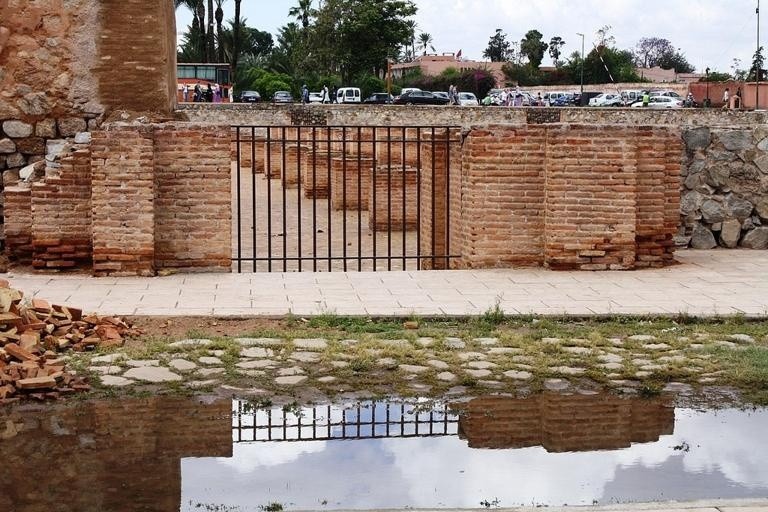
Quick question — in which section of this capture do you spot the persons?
[448,82,549,109]
[643,91,650,107]
[301,84,338,104]
[687,92,695,108]
[736,87,741,108]
[183,81,220,103]
[723,88,729,109]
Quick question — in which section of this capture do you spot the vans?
[456,92,479,106]
[333,87,362,104]
[400,88,422,96]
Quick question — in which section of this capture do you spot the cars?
[267,91,294,104]
[306,91,324,104]
[364,92,395,104]
[509,89,686,109]
[394,90,451,105]
[237,91,262,103]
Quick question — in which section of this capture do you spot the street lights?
[575,32,585,94]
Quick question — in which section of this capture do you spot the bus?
[176,61,233,103]
